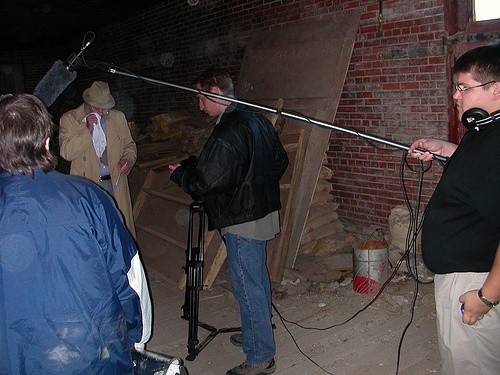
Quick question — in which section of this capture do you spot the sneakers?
[231,333,243,346]
[225,358,276,375]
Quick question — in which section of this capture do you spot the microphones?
[33,42,89,108]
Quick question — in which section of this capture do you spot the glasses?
[451,80,496,92]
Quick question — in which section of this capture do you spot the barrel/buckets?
[353,227,388,295]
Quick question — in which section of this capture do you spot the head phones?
[462,108,500,132]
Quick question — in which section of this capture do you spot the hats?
[82,81,115,108]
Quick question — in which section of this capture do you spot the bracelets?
[479,289,499,309]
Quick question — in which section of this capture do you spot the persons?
[167,72,289,375]
[0,93,153,375]
[58,81,137,240]
[407,44,500,375]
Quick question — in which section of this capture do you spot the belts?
[100,175,111,181]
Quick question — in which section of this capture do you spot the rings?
[126,162,132,168]
